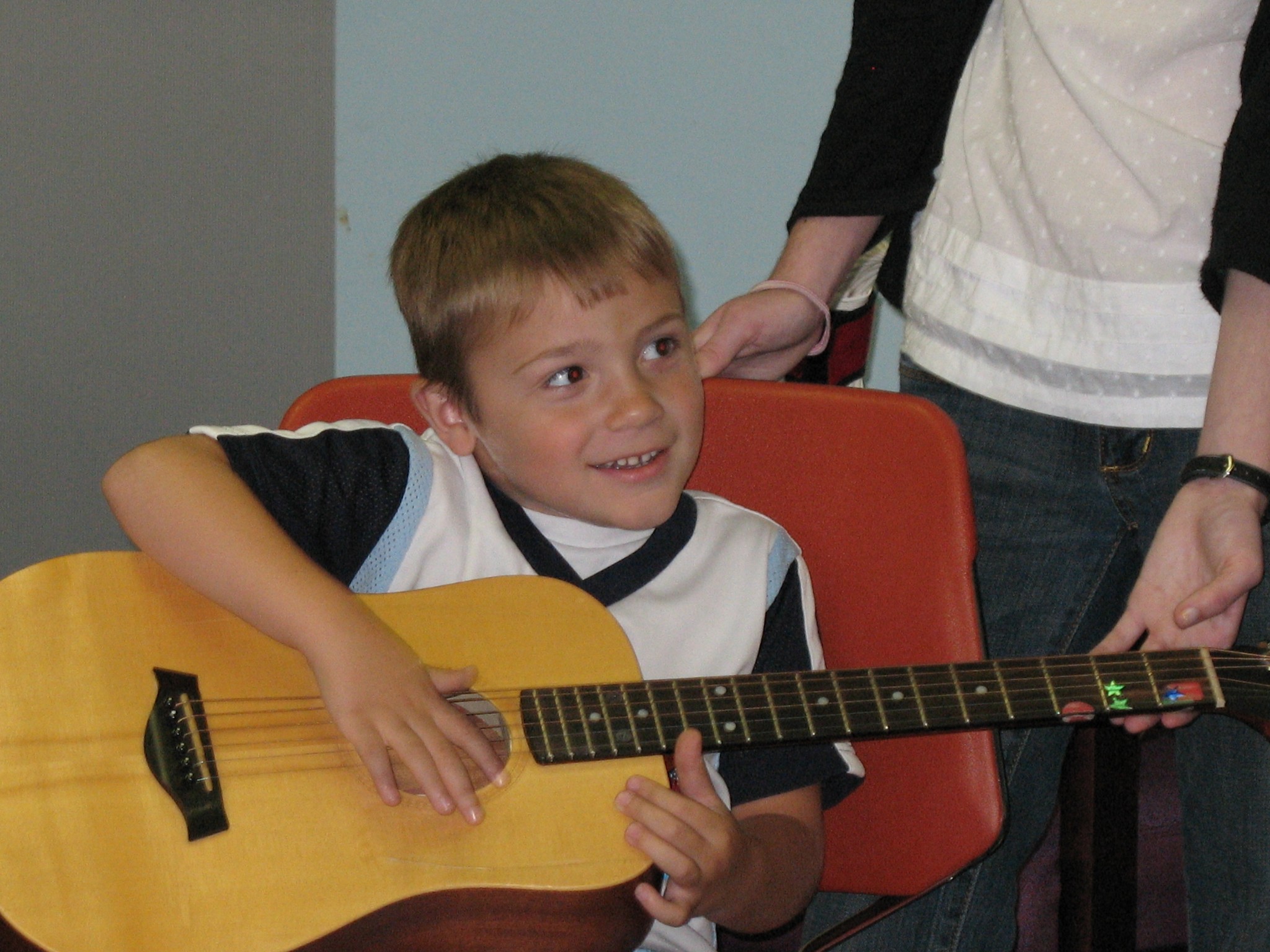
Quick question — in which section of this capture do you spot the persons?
[691,0,1270,952]
[104,154,866,952]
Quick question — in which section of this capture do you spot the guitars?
[0,549,1267,952]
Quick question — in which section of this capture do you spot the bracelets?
[1178,453,1270,497]
[750,280,831,356]
